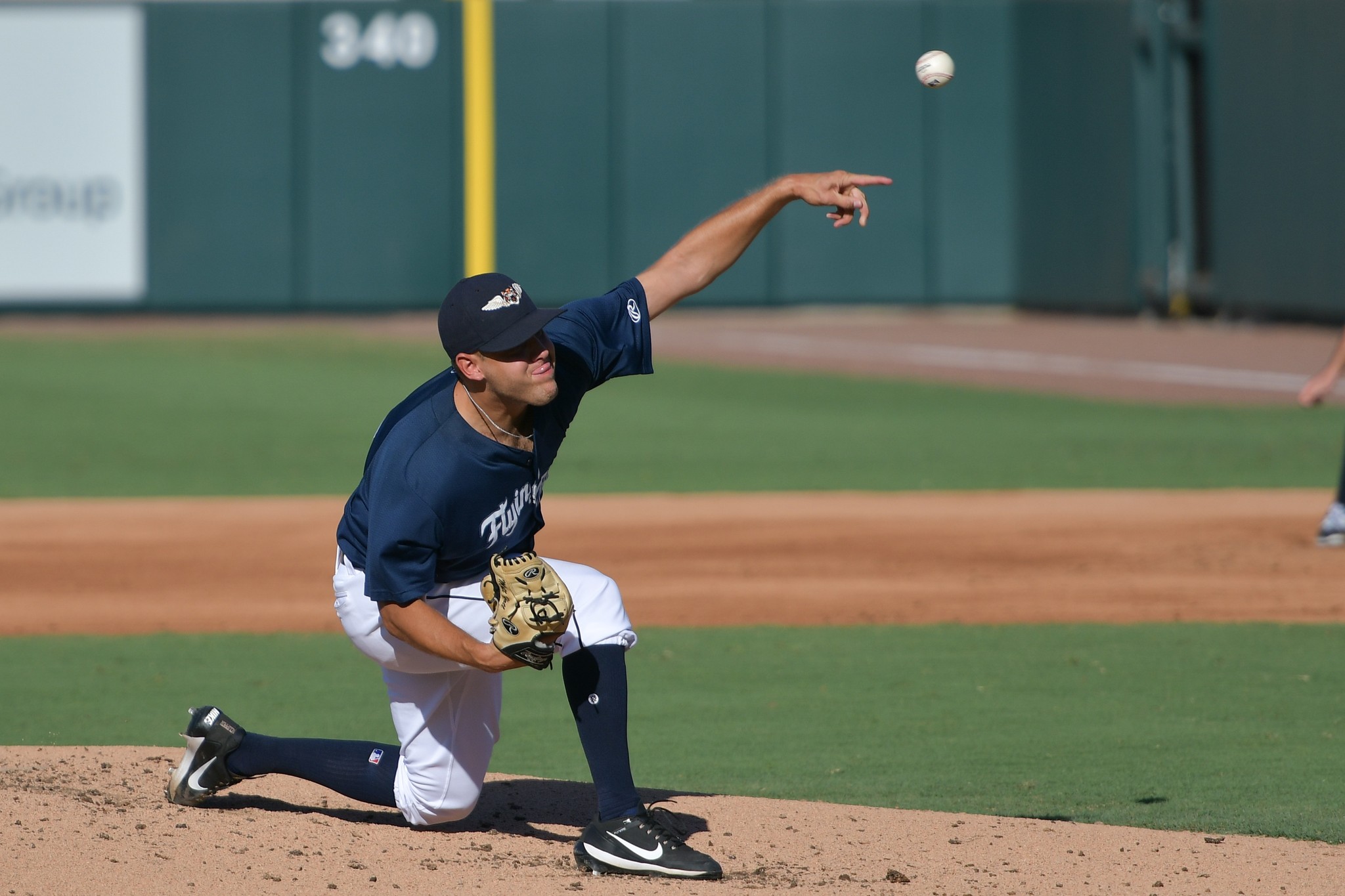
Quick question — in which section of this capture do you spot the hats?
[438,272,569,360]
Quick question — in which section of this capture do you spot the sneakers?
[168,706,245,808]
[572,798,724,880]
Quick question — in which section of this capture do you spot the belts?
[338,552,345,566]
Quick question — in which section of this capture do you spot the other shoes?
[1314,502,1345,545]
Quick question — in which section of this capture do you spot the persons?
[168,167,891,879]
[1296,334,1345,548]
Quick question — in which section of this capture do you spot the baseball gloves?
[479,551,574,671]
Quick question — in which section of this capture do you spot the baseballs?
[914,49,956,87]
